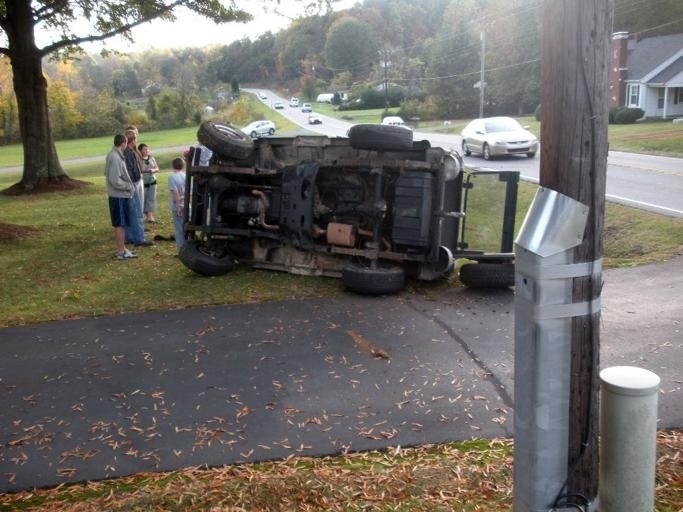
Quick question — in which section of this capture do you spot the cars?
[178,114,520,293]
[257,90,312,112]
[308,115,323,125]
[461,116,538,160]
[241,120,276,139]
[382,116,405,127]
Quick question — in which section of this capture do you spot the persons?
[121,130,156,248]
[123,124,149,232]
[194,138,212,168]
[165,157,193,257]
[179,147,197,226]
[136,143,160,223]
[102,133,139,261]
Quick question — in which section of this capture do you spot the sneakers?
[147,218,156,223]
[124,238,156,247]
[113,248,138,260]
